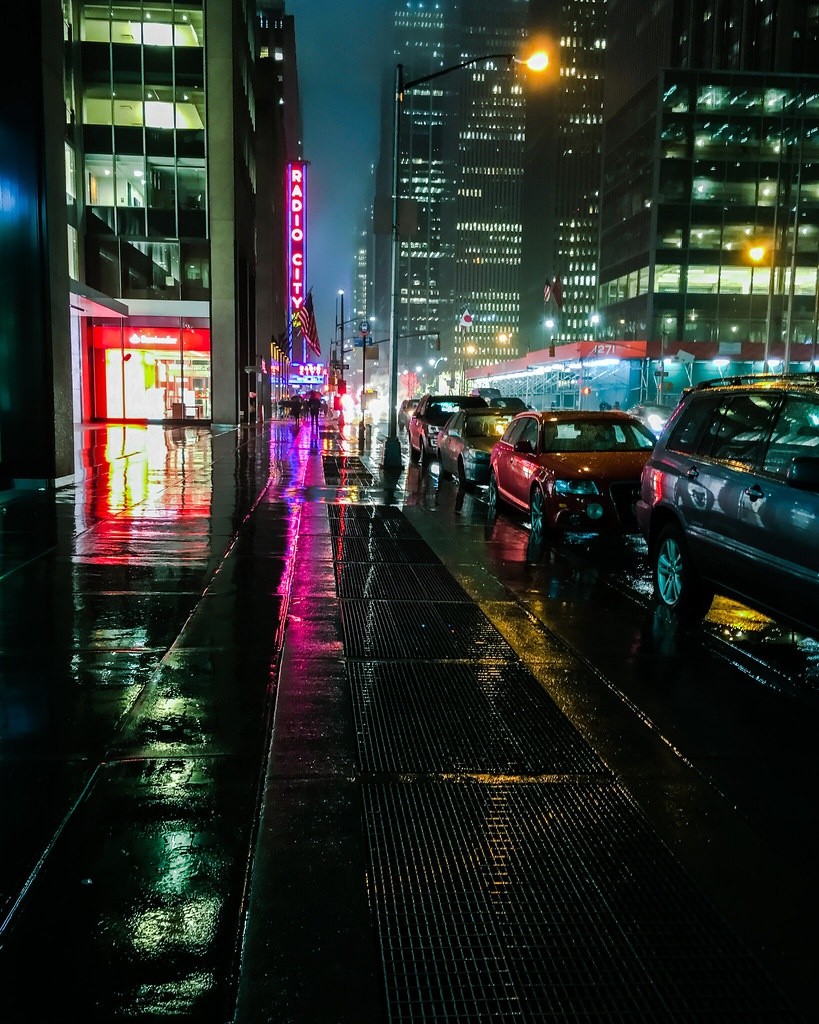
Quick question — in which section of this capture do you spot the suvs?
[408,393,489,468]
[637,371,819,646]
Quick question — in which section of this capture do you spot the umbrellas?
[305,390,323,399]
[250,392,257,398]
[291,394,304,400]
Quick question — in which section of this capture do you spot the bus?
[469,388,502,403]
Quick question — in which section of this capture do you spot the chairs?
[572,426,597,450]
[534,423,558,451]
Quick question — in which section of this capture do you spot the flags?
[551,275,563,308]
[459,310,473,327]
[543,278,551,303]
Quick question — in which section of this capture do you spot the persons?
[308,398,321,426]
[290,398,302,425]
[303,398,309,422]
[598,399,609,411]
[323,401,328,417]
[549,401,555,411]
[250,398,256,421]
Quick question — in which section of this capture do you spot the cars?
[437,407,529,487]
[486,397,529,415]
[623,403,677,439]
[488,411,658,547]
[398,399,421,431]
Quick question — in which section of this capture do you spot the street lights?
[358,330,441,453]
[741,236,779,380]
[325,290,377,420]
[381,43,552,472]
